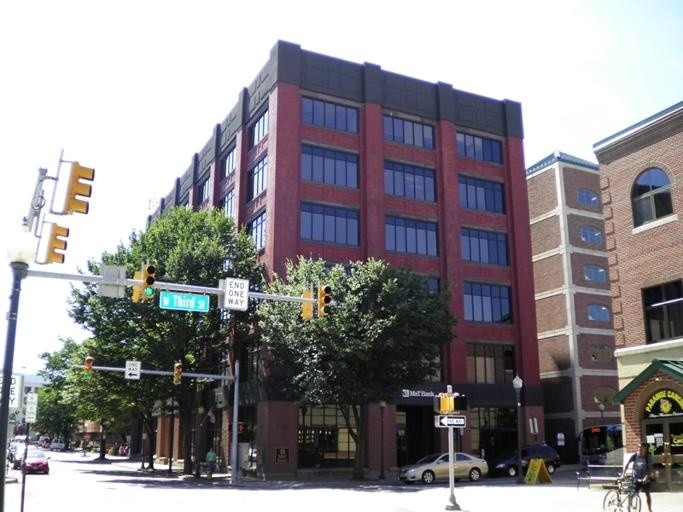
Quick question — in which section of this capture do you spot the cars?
[5,431,66,474]
[399,451,489,485]
[298,447,324,468]
[487,445,561,477]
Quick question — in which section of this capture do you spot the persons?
[205,447,217,480]
[619,442,653,512]
[10,438,17,458]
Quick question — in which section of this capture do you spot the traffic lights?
[143,264,155,298]
[237,421,246,433]
[173,362,182,385]
[83,356,94,379]
[432,392,448,413]
[45,223,68,264]
[62,162,94,215]
[319,284,332,319]
[450,395,467,412]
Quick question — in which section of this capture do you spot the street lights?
[378,395,386,481]
[512,375,524,484]
[598,403,605,425]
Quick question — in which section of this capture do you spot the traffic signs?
[437,414,467,429]
[222,278,251,314]
[24,392,38,424]
[157,289,210,314]
[123,360,142,381]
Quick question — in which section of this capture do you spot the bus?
[578,424,623,470]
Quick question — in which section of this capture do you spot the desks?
[587,463,624,490]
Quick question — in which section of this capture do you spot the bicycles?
[602,471,650,511]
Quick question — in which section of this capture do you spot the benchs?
[574,472,622,492]
